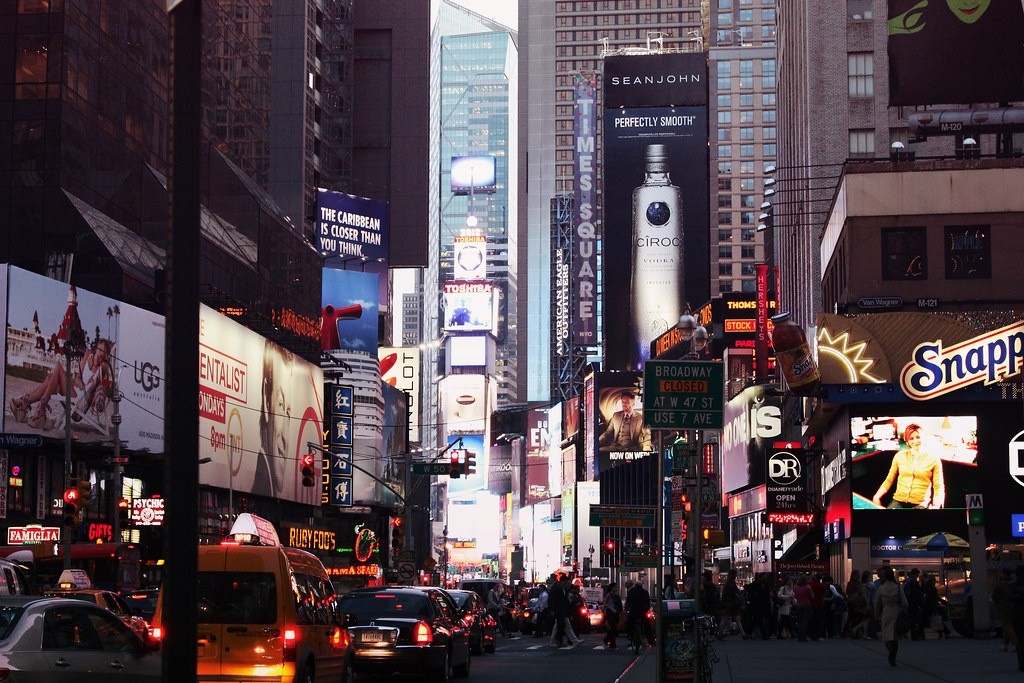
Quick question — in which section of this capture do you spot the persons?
[873,571,909,667]
[9,338,112,428]
[487,574,656,651]
[249,338,293,499]
[662,564,1024,673]
[873,424,944,510]
[598,392,652,452]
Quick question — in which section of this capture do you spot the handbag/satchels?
[896,611,913,634]
[847,591,867,614]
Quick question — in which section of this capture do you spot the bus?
[0,543,145,595]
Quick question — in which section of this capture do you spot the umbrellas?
[905,531,970,585]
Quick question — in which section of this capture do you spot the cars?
[335,586,471,683]
[33,562,61,595]
[57,569,92,589]
[520,598,604,636]
[43,590,149,649]
[0,560,32,595]
[446,589,498,655]
[0,595,160,683]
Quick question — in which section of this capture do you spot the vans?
[146,544,354,682]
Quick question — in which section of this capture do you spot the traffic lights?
[449,452,460,478]
[302,455,315,487]
[392,517,404,548]
[608,543,614,549]
[465,452,476,475]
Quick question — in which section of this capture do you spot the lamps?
[764,176,840,187]
[763,186,837,197]
[963,138,976,159]
[760,199,833,210]
[198,283,352,374]
[763,163,844,174]
[758,212,828,222]
[757,223,824,232]
[892,142,905,162]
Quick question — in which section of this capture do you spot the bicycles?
[625,611,642,656]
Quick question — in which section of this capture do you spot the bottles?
[770,311,821,393]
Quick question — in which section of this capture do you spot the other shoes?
[717,635,724,641]
[777,635,786,640]
[573,639,584,646]
[742,635,752,640]
